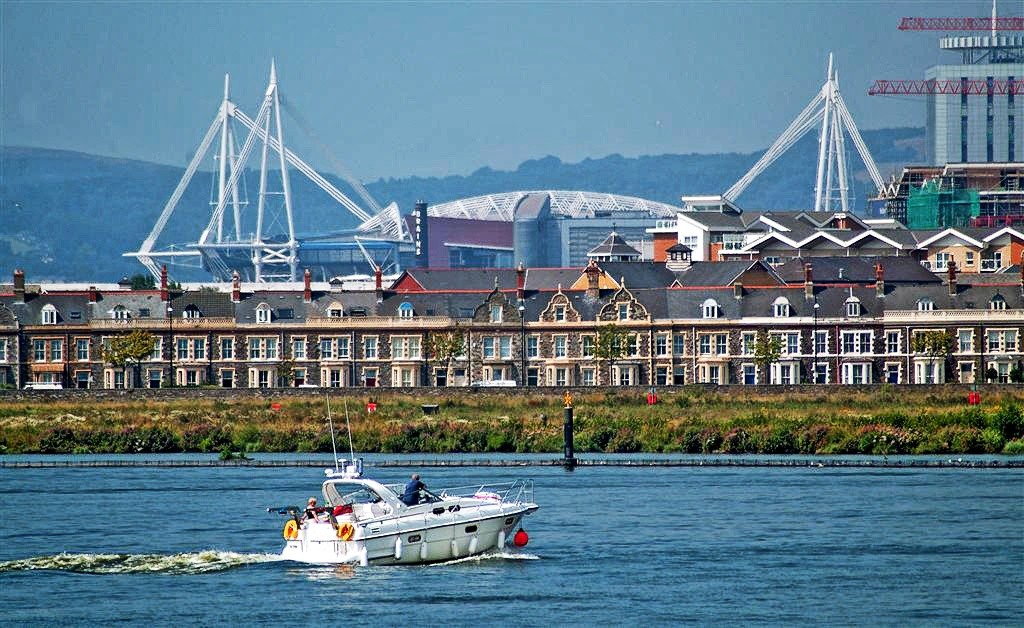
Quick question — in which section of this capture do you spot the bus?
[22,381,63,391]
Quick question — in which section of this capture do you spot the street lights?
[518,295,527,388]
[812,296,821,386]
[167,293,176,389]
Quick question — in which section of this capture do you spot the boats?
[266,390,540,569]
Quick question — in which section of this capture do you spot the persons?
[402,474,424,506]
[300,497,320,523]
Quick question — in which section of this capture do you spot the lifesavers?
[335,524,355,541]
[283,519,298,541]
